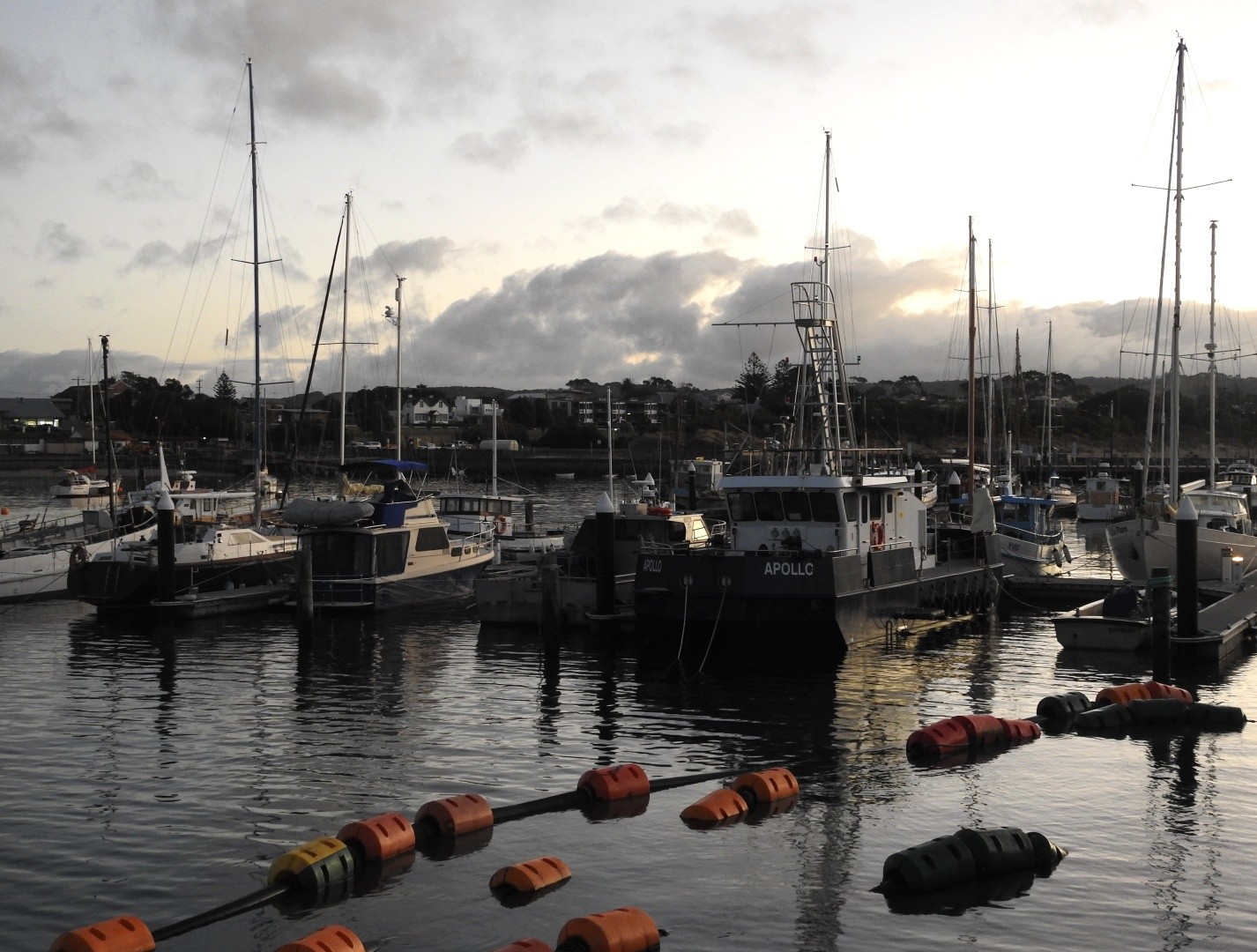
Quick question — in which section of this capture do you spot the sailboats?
[1,57,563,623]
[525,31,1256,655]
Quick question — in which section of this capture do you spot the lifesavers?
[493,514,507,534]
[871,523,884,550]
[69,545,86,570]
